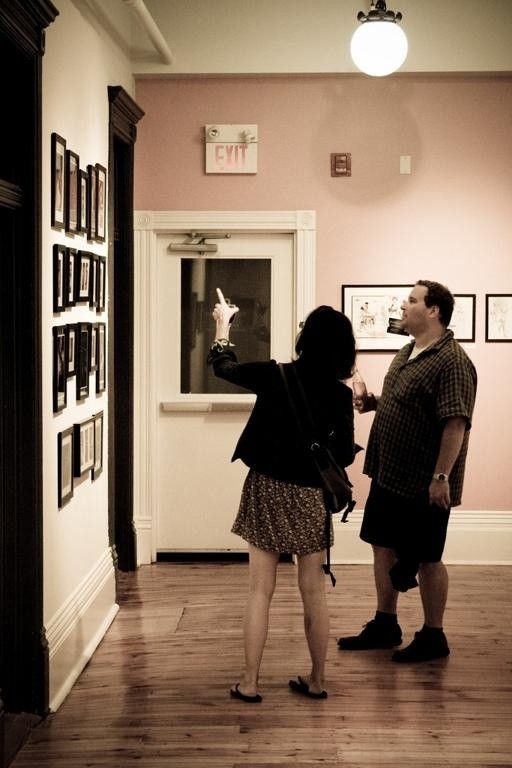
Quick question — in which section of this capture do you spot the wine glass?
[223,305,237,346]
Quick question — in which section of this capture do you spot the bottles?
[350,365,368,398]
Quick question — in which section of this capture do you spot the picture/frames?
[448,291,479,342]
[341,280,418,352]
[48,130,107,510]
[484,290,512,345]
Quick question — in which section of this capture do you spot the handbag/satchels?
[310,435,353,513]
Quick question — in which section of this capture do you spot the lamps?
[350,0,410,80]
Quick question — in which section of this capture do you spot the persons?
[338,281,476,666]
[210,286,362,706]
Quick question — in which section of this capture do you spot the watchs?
[430,471,451,483]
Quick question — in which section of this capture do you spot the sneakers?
[337,620,402,649]
[392,632,449,663]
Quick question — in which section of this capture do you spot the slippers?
[230,683,262,703]
[289,675,327,699]
